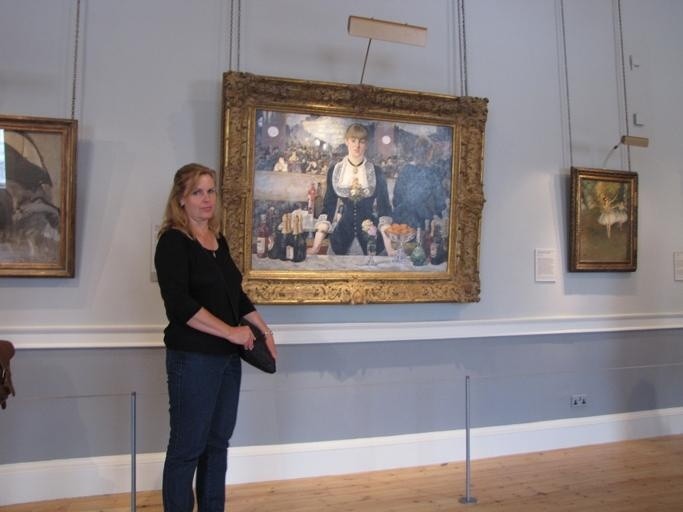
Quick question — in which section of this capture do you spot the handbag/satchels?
[235,318,276,376]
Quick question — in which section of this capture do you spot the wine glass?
[383,230,416,266]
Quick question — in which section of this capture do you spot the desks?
[254,170,394,203]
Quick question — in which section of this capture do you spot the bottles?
[255,206,306,263]
[307,183,315,214]
[315,183,323,218]
[408,199,448,267]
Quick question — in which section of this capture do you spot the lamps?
[603,136,649,167]
[347,17,426,84]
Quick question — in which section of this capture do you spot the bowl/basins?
[306,246,328,255]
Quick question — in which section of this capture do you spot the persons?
[152,163,278,512]
[597,189,620,240]
[273,122,446,268]
[613,200,628,231]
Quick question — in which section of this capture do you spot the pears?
[410,246,427,267]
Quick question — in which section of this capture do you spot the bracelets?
[262,331,273,336]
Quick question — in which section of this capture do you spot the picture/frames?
[220,72,486,303]
[570,166,637,272]
[0,113,78,278]
